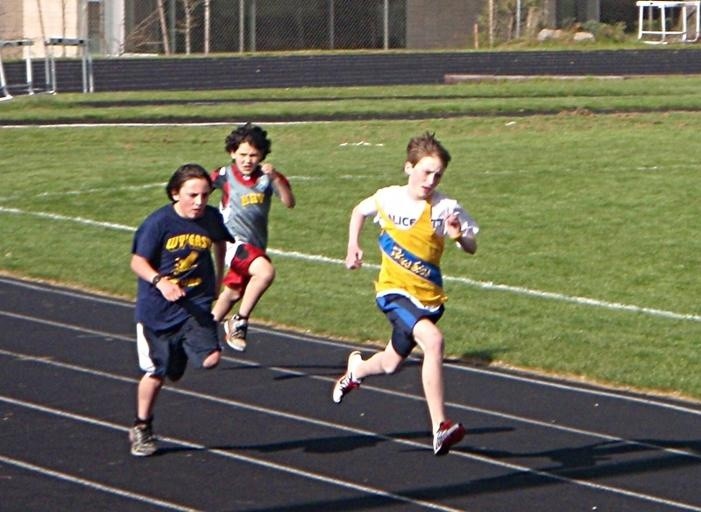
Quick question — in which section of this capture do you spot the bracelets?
[152,273,163,286]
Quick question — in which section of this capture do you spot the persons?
[332,129,481,459]
[208,119,294,352]
[130,164,236,457]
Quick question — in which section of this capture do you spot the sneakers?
[331,351,365,404]
[433,419,466,458]
[224,315,248,351]
[127,422,158,458]
